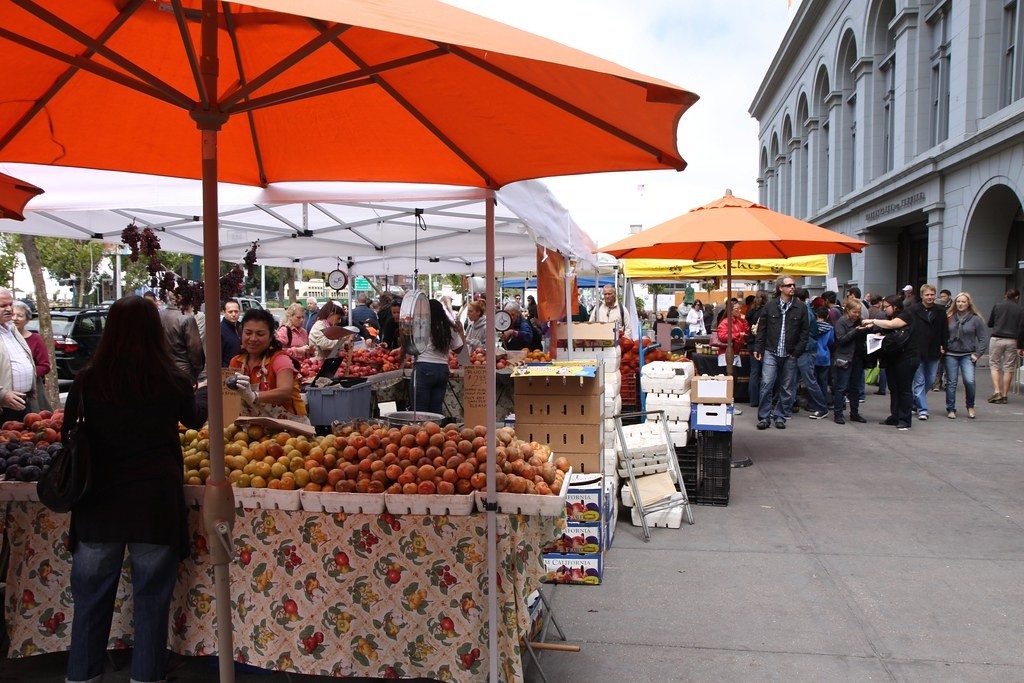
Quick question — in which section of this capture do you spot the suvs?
[23,306,109,382]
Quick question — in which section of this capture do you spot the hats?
[902,285,912,291]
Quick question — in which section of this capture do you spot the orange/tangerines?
[519,348,552,363]
[619,331,689,374]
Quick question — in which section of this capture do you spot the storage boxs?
[1,320,734,683]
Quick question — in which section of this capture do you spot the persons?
[0,274,1024,682]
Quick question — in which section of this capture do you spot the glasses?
[781,283,796,288]
[516,298,521,300]
[528,299,531,302]
[884,304,891,309]
[847,295,851,298]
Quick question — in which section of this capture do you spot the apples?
[181,424,337,491]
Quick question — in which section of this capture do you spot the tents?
[597,252,619,305]
[499,275,614,309]
[0,160,598,361]
[622,253,832,305]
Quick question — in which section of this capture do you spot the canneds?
[702,345,711,355]
[739,349,750,356]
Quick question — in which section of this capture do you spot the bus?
[283,278,349,310]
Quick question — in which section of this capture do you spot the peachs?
[0,409,65,449]
[310,422,569,494]
[297,343,415,382]
[448,348,507,369]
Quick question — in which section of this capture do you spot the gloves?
[235,372,259,406]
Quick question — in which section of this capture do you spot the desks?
[301,369,402,413]
[0,503,571,683]
[404,366,514,420]
[693,353,762,404]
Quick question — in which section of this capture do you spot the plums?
[0,437,64,482]
[225,375,240,390]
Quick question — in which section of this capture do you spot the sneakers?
[770,406,798,419]
[808,411,829,419]
[988,392,1009,404]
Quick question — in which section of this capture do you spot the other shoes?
[844,395,865,403]
[734,408,742,415]
[757,420,769,429]
[948,412,956,419]
[967,408,976,418]
[850,415,867,423]
[879,419,911,428]
[933,388,940,392]
[774,417,785,429]
[834,416,845,424]
[911,408,929,419]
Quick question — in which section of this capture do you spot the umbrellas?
[0,0,700,681]
[0,172,44,221]
[590,188,871,375]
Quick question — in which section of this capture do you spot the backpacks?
[520,320,543,352]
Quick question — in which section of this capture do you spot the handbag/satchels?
[751,317,760,336]
[710,332,733,349]
[882,327,911,353]
[36,365,93,514]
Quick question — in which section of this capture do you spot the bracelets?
[253,390,259,404]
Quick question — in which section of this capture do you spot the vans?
[232,297,264,317]
